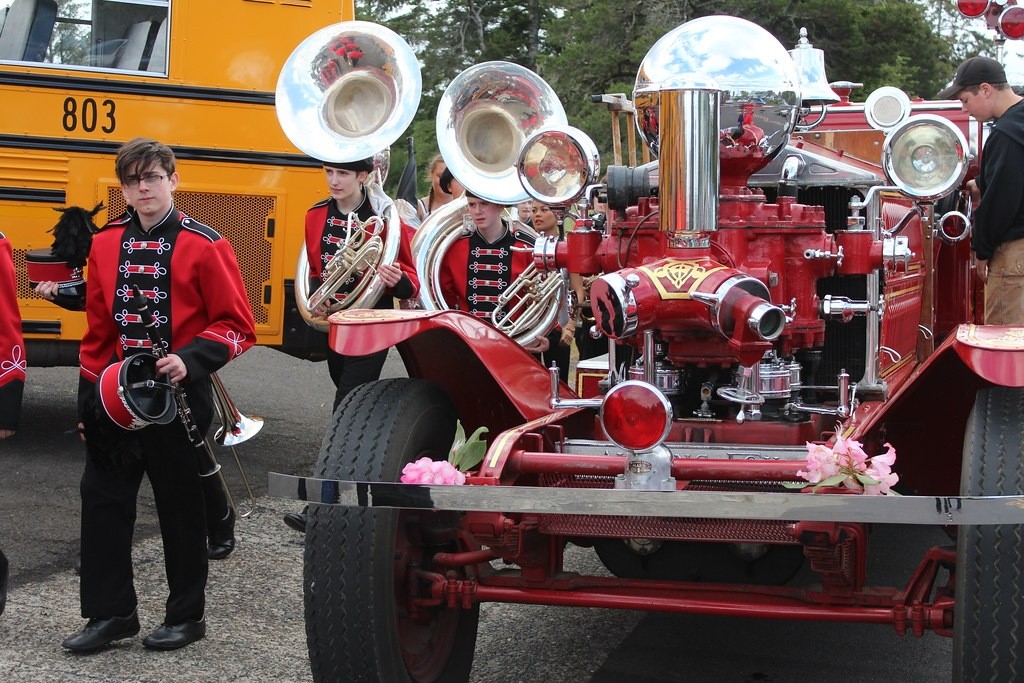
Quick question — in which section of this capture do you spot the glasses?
[120,173,171,189]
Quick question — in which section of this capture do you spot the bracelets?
[563,324,575,335]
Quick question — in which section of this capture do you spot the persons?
[640,107,661,140]
[737,110,754,127]
[328,34,365,68]
[0,231,25,616]
[937,56,1024,327]
[283,150,618,532]
[35,138,259,653]
[521,109,544,131]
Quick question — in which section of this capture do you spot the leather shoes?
[61,604,141,649]
[143,606,206,650]
[283,501,309,531]
[208,506,235,559]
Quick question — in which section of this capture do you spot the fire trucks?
[267,1,1024,683]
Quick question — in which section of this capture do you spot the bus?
[0,0,355,366]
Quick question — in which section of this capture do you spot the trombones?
[210,371,265,521]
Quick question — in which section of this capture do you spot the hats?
[466,191,512,208]
[936,56,1006,99]
[323,157,374,173]
[439,165,454,195]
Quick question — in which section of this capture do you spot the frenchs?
[409,59,573,352]
[272,18,425,335]
